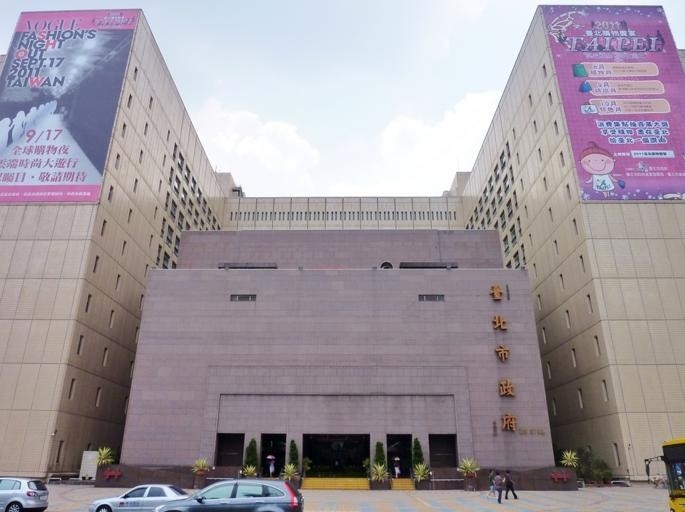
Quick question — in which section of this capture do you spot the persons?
[264,458,270,478]
[505,469,518,500]
[493,472,506,505]
[392,460,401,479]
[269,458,275,478]
[486,468,496,499]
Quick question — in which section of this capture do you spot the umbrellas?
[391,455,400,462]
[265,455,276,460]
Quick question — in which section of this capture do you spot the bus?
[661,438,685,511]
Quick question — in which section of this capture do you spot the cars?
[154,481,304,512]
[89,483,190,512]
[0,477,49,512]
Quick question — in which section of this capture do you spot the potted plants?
[592,467,613,486]
[191,456,210,477]
[457,456,479,478]
